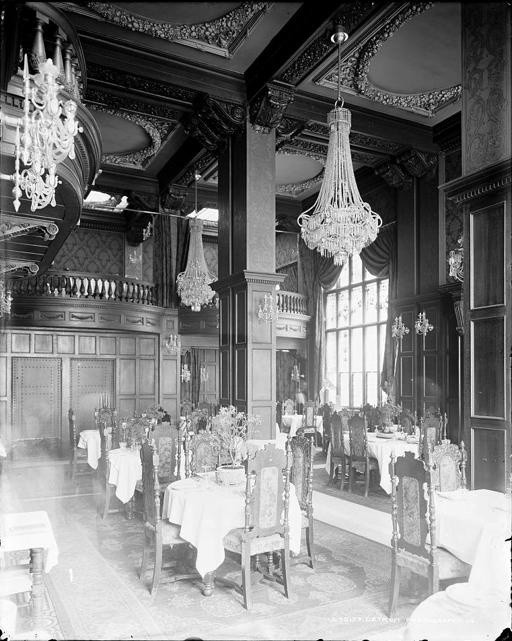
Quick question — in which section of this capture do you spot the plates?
[171,482,201,490]
[242,474,248,479]
[109,449,120,453]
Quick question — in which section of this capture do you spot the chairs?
[98,419,136,519]
[223,442,289,610]
[274,396,377,456]
[138,438,202,600]
[386,451,469,617]
[288,436,316,568]
[68,408,97,487]
[427,439,467,490]
[326,401,446,492]
[348,412,378,497]
[100,401,275,504]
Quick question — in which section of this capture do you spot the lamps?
[182,364,191,384]
[412,310,435,335]
[165,334,183,356]
[177,170,224,315]
[295,24,384,268]
[390,315,411,340]
[0,1,86,214]
[257,293,278,324]
[448,236,465,284]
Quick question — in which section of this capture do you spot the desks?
[163,469,302,595]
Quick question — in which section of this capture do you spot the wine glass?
[374,425,419,443]
[118,441,127,455]
[201,465,212,490]
